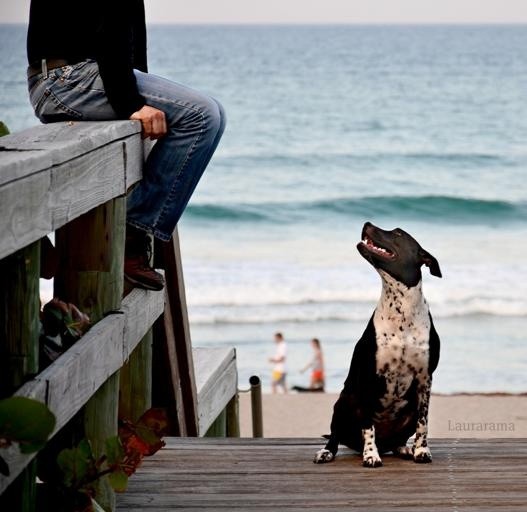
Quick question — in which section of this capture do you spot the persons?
[26,0,227,292]
[268,332,288,394]
[299,338,325,390]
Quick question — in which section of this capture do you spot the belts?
[27,59,83,79]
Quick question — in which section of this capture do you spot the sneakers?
[126,227,165,290]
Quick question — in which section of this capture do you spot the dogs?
[311,220,444,470]
